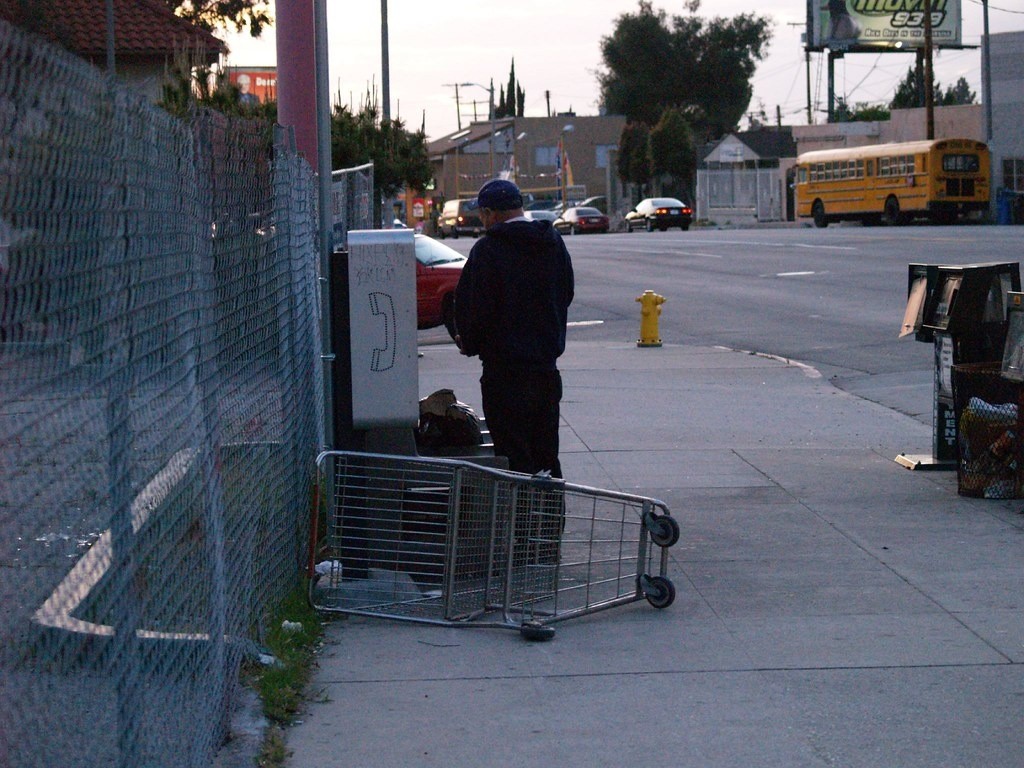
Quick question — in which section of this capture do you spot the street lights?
[489,132,501,181]
[514,132,528,185]
[461,82,498,183]
[560,126,573,213]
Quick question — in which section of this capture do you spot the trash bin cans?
[893,260,1024,500]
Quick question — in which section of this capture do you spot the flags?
[558,140,575,193]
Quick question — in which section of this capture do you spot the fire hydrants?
[634,289,667,349]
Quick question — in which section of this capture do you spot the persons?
[453,181,575,567]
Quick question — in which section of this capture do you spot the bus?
[792,137,992,226]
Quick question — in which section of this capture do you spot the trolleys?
[305,450,682,644]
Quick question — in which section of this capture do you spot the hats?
[467,179,523,211]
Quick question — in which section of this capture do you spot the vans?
[436,197,491,239]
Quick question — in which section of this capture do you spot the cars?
[411,231,470,329]
[550,206,609,235]
[521,195,608,221]
[623,196,694,231]
